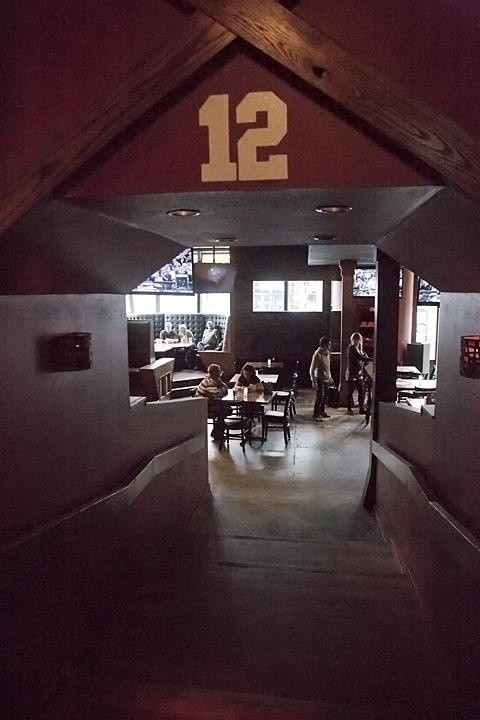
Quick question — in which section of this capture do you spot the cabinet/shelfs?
[354,303,375,348]
[407,343,430,374]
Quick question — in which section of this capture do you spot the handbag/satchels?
[261,382,273,391]
[329,386,340,408]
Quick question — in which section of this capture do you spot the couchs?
[126,313,233,374]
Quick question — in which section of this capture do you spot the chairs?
[190,358,299,453]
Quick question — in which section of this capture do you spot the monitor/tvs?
[129,246,196,296]
[417,277,440,306]
[352,264,404,299]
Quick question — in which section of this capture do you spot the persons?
[177,324,194,342]
[160,322,177,343]
[234,365,260,427]
[194,321,221,370]
[196,364,232,442]
[309,337,334,422]
[345,332,374,415]
